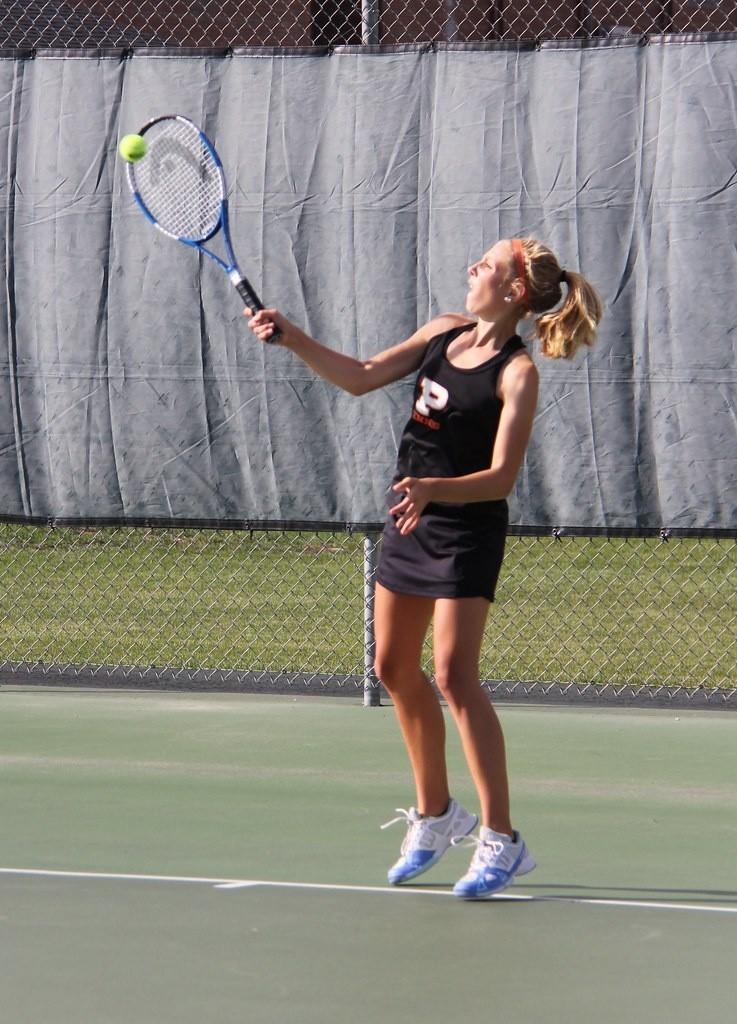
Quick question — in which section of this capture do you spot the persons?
[244,236,602,900]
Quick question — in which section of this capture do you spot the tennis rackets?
[126,114,284,345]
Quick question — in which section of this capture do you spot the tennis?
[118,134,148,163]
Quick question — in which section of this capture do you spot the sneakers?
[379,797,481,884]
[449,828,538,901]
